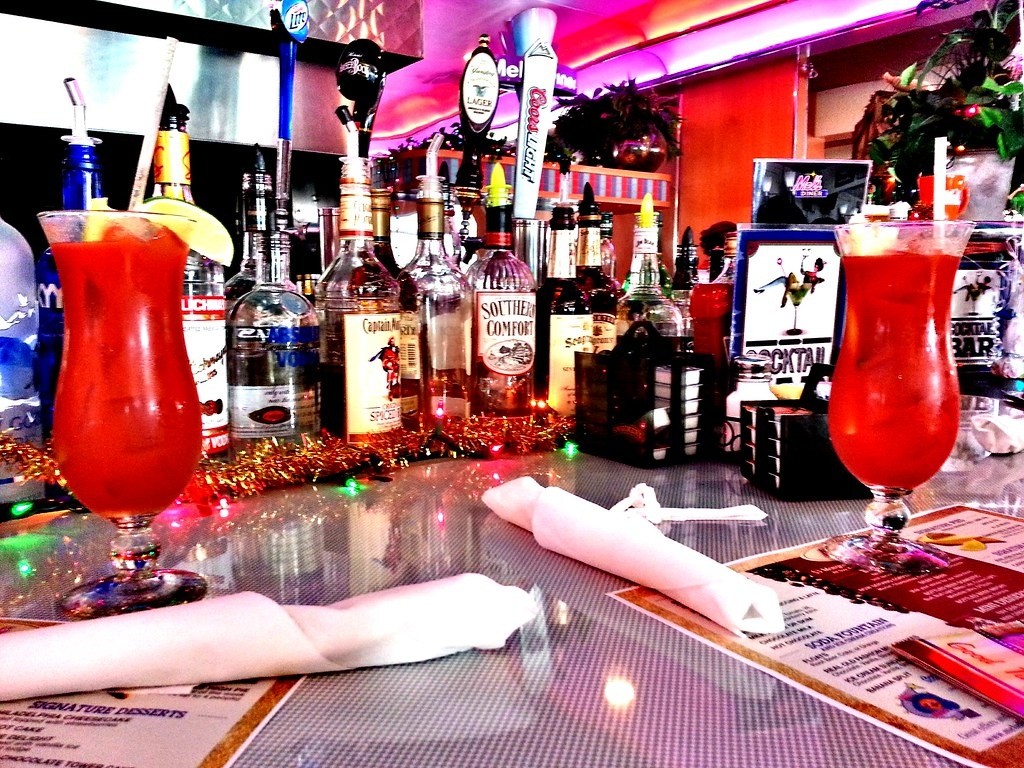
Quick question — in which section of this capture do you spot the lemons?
[139,196,233,267]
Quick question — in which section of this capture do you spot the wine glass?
[829,217,977,573]
[37,207,209,619]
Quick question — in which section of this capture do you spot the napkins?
[969,414,1024,457]
[482,476,786,634]
[0,574,536,702]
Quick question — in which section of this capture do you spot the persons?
[383,336,400,388]
[953,276,991,301]
[753,258,827,308]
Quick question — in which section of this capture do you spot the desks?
[0,440,1024,768]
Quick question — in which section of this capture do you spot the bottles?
[36,143,112,447]
[317,156,402,456]
[221,229,323,460]
[534,202,739,434]
[296,273,322,304]
[463,184,535,419]
[369,186,422,430]
[442,208,462,267]
[398,175,471,436]
[135,100,231,467]
[224,172,275,339]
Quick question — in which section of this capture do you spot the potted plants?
[861,0,1024,221]
